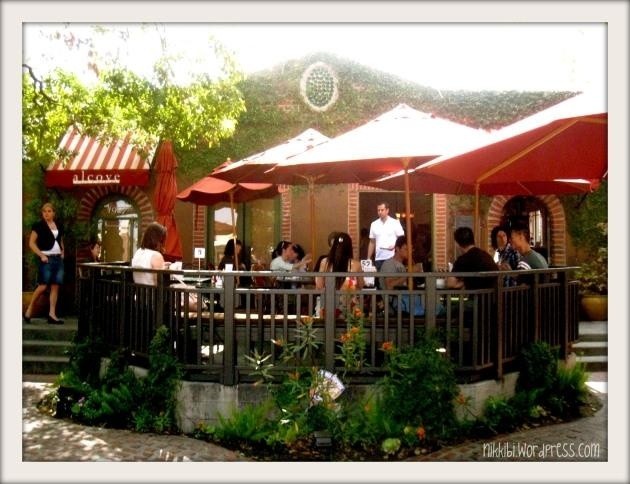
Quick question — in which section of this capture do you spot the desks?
[175,273,461,314]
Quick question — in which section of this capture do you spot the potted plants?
[565,177,608,319]
[22,171,97,318]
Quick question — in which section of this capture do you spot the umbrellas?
[209,126,331,267]
[368,79,607,252]
[175,154,278,284]
[263,103,486,287]
[153,139,177,247]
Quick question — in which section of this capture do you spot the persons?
[23,203,65,324]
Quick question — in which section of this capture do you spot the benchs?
[177,306,458,383]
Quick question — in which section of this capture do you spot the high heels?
[47,315,65,324]
[23,312,31,324]
